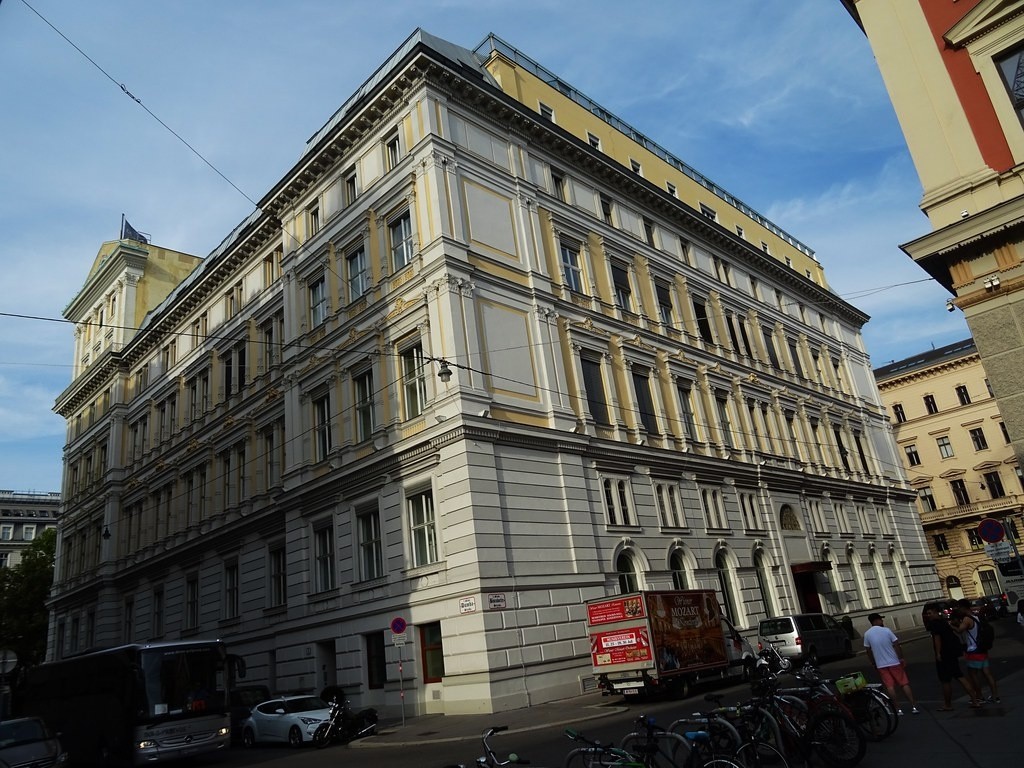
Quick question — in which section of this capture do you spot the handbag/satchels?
[977,624,996,651]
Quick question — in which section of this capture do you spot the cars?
[0,716,70,768]
[242,695,333,749]
[922,597,958,631]
[965,593,1009,624]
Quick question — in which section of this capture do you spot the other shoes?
[897,708,905,715]
[912,704,921,714]
[969,696,1002,708]
[936,706,954,711]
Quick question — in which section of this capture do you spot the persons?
[927,599,1024,709]
[863,614,921,715]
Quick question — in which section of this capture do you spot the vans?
[757,611,852,663]
[230,685,273,735]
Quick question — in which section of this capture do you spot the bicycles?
[444,645,900,768]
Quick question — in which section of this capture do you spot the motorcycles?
[755,634,793,671]
[313,696,379,749]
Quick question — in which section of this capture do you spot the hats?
[868,613,885,621]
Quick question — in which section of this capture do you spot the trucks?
[586,587,757,700]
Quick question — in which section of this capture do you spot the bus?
[17,638,247,768]
